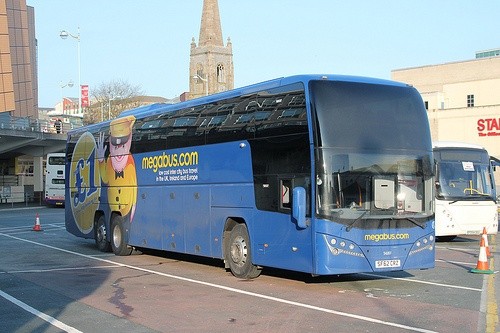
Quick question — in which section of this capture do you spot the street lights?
[61,26,82,113]
[108,96,120,120]
[192,75,209,95]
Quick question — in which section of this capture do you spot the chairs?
[0,185,14,207]
[23,184,41,207]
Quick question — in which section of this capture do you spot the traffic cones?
[470,228,494,274]
[32,213,44,232]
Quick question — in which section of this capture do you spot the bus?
[44,152,66,208]
[64,74,441,280]
[431,141,500,242]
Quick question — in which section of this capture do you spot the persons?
[54,117,63,134]
[26,166,33,176]
